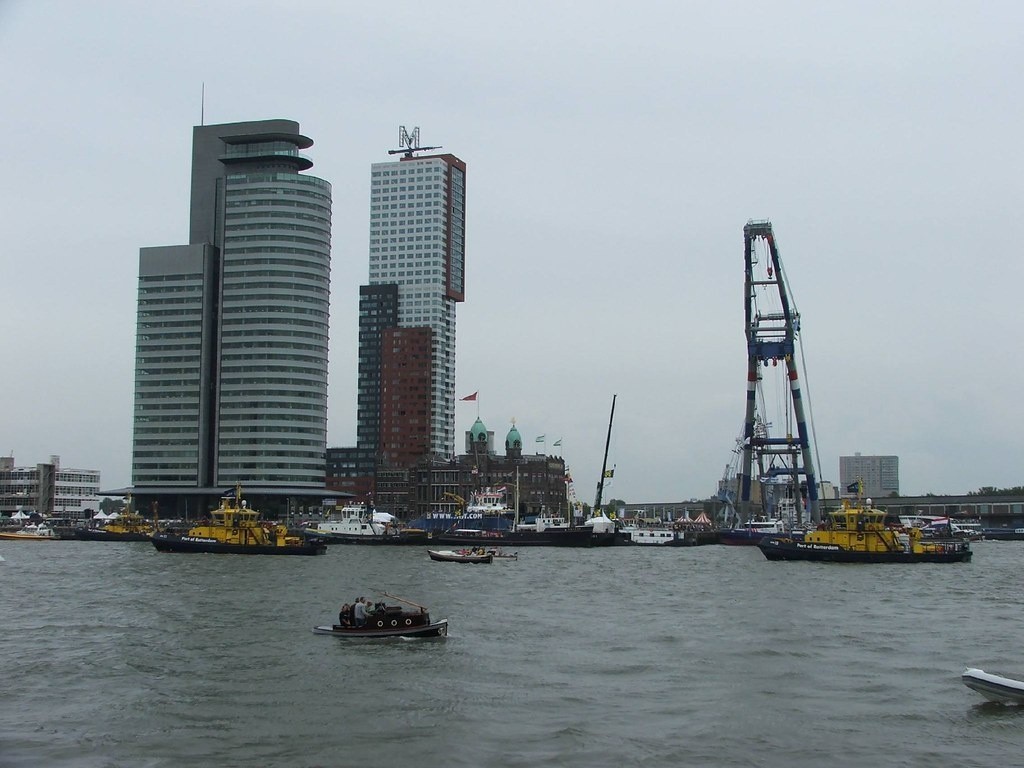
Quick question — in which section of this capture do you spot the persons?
[461,545,502,556]
[339,597,374,628]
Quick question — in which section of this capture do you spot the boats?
[149,482,329,557]
[756,480,975,567]
[427,545,494,565]
[961,667,1024,708]
[312,587,450,641]
[72,489,166,542]
[0,521,63,539]
[898,509,1023,541]
[434,464,596,550]
[303,500,439,546]
